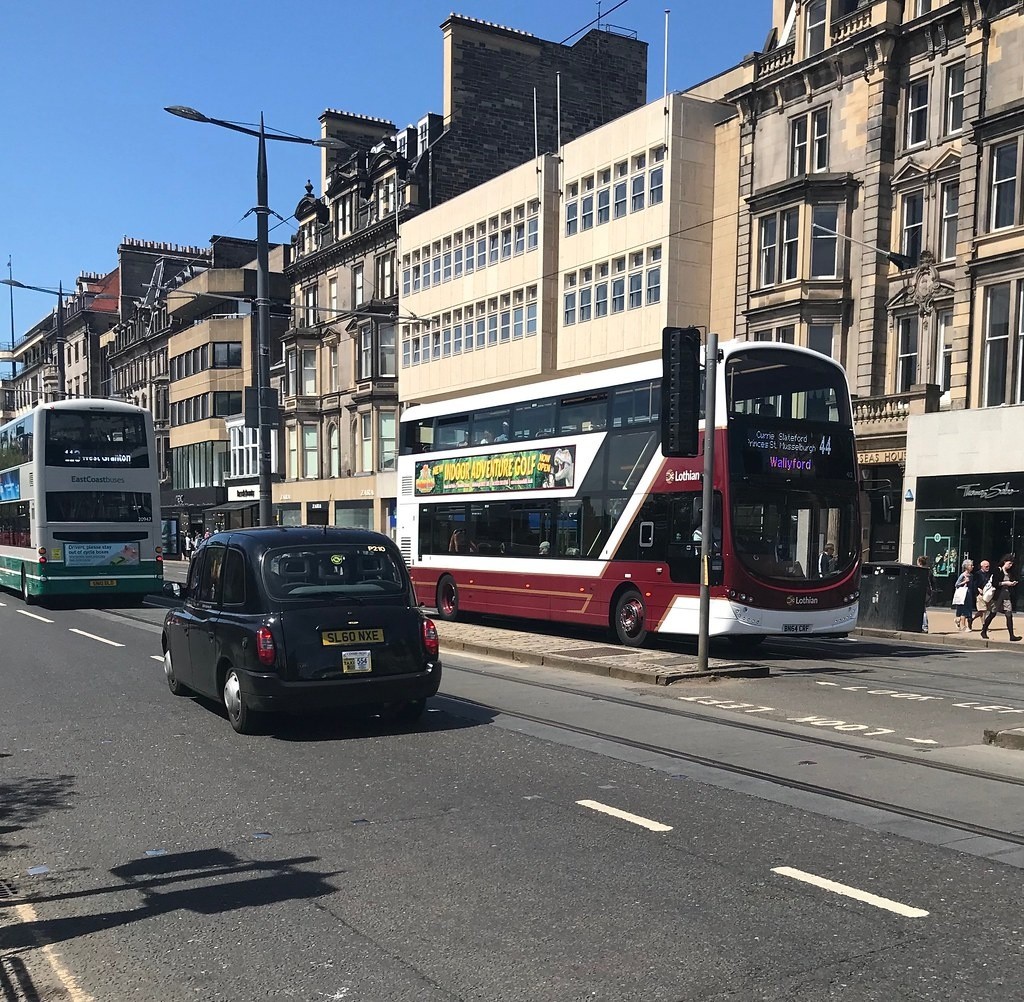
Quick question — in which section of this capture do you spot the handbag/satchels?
[952,586,968,604]
[982,582,995,602]
[975,593,988,611]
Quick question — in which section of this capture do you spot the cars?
[160,524,443,736]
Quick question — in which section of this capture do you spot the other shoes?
[923,629,928,633]
[968,618,972,629]
[1010,635,1022,641]
[954,618,960,629]
[961,626,967,631]
[981,631,989,639]
[965,628,971,633]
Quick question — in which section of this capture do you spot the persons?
[458,431,468,447]
[981,554,1023,641]
[494,421,510,443]
[968,560,991,632]
[955,559,974,632]
[692,506,744,570]
[180,520,240,562]
[917,556,935,634]
[818,543,835,578]
[481,431,493,444]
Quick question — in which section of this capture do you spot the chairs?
[356,555,385,580]
[277,557,311,583]
[318,562,352,586]
[448,528,493,552]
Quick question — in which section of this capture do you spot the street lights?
[169,100,358,528]
[0,275,121,398]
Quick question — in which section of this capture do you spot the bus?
[395,341,864,644]
[0,400,167,605]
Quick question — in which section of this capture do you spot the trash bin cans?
[856,562,929,633]
